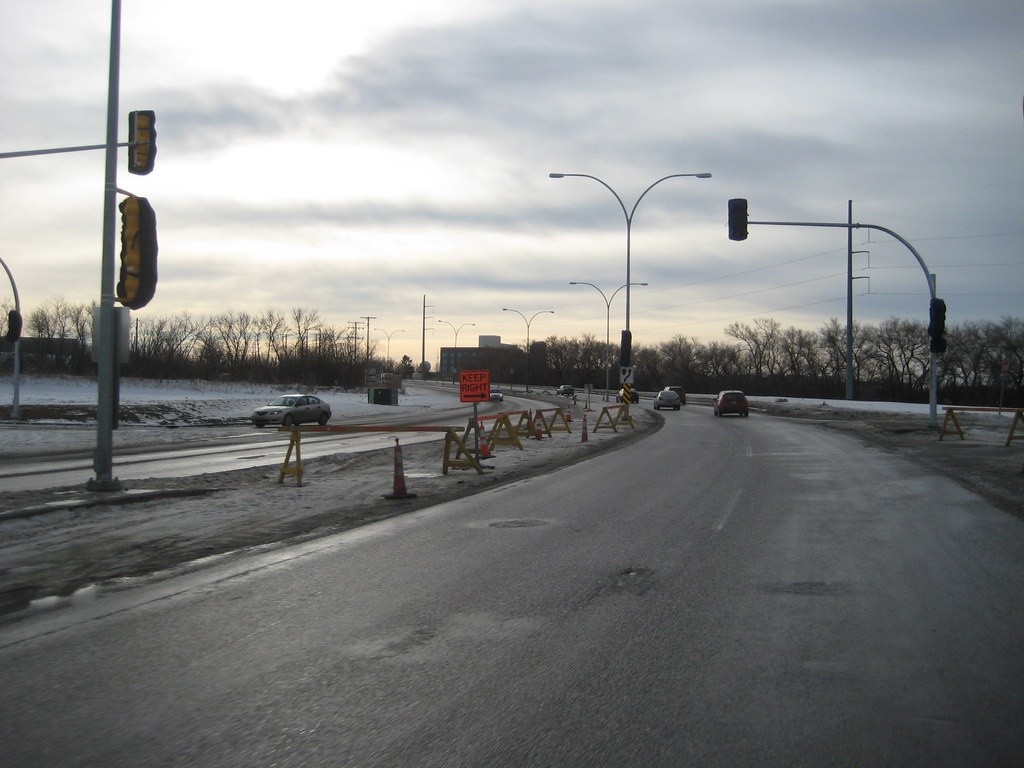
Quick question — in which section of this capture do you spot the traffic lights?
[928,298,947,338]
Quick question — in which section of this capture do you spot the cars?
[652,391,682,410]
[251,394,331,427]
[616,389,640,403]
[556,385,574,396]
[713,391,748,417]
[663,386,686,405]
[489,388,503,401]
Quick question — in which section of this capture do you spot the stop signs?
[1001,362,1009,373]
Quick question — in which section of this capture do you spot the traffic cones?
[524,405,590,442]
[479,421,496,459]
[380,438,417,499]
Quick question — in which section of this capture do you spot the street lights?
[503,308,554,392]
[374,328,405,358]
[550,173,712,421]
[438,320,475,384]
[569,282,648,402]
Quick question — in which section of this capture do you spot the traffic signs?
[459,370,490,403]
